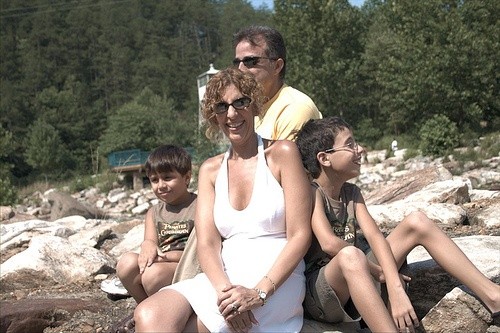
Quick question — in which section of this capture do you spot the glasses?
[316,138,359,153]
[214,97,252,114]
[232,56,278,68]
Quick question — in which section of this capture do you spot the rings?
[232,304,236,310]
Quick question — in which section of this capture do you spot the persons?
[233,27,322,142]
[294,118,500,333]
[133,66,312,333]
[116,145,196,297]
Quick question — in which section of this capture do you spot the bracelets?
[265,275,275,295]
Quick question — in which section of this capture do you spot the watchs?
[255,288,266,306]
